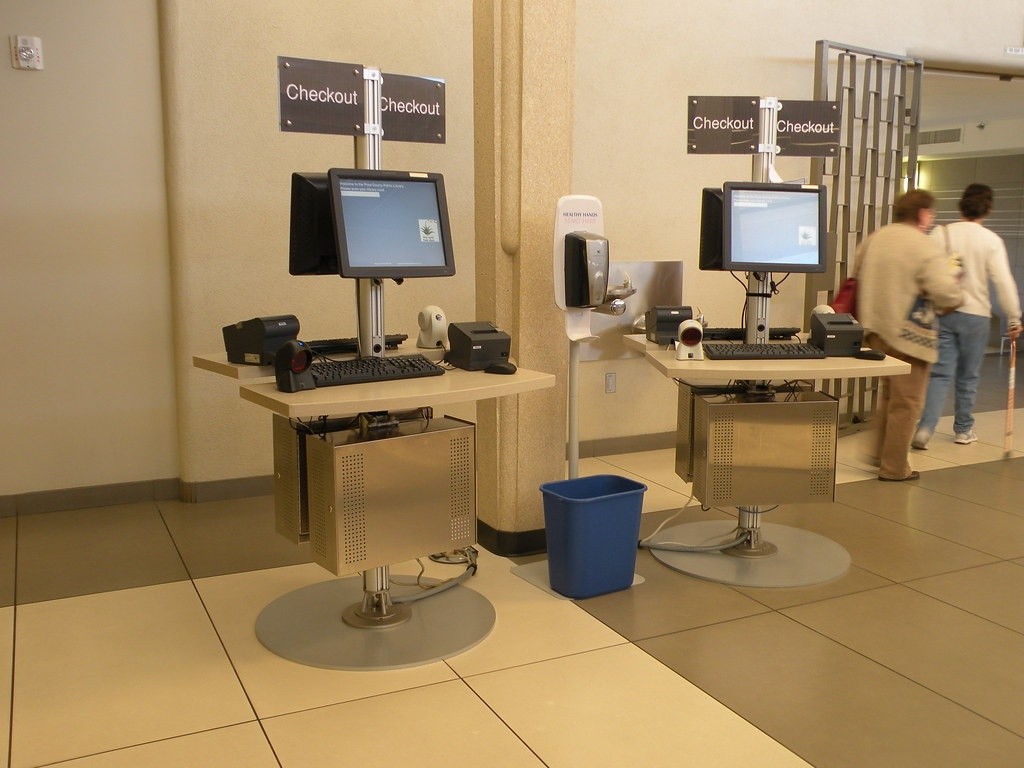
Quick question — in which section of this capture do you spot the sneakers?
[911,427,931,450]
[954,429,978,443]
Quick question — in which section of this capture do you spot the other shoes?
[878,471,920,481]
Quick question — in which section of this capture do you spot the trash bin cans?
[538,473,648,600]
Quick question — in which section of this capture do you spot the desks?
[623,332,911,587]
[238,361,556,629]
[191,339,452,543]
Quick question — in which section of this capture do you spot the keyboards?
[311,354,444,387]
[305,334,408,355]
[702,342,827,360]
[701,327,801,341]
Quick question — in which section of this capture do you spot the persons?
[847,187,967,482]
[910,183,1024,449]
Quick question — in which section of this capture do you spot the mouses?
[484,362,517,374]
[855,350,886,360]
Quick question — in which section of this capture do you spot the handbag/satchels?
[829,231,874,318]
[931,224,966,316]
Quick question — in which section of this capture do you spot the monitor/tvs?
[698,182,827,273]
[289,167,456,276]
